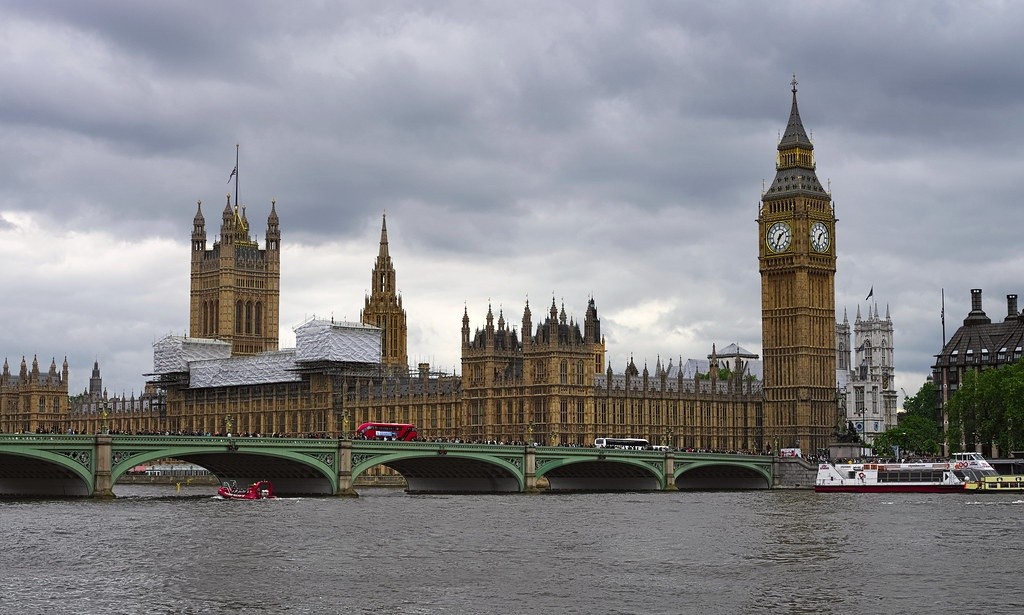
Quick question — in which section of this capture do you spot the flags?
[865,286,873,300]
[227,166,237,183]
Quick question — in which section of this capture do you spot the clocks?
[810,222,830,253]
[765,221,792,254]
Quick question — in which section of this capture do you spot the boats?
[964,475,1024,493]
[812,452,1000,493]
[217,480,277,499]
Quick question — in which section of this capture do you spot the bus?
[595,438,650,450]
[356,422,417,442]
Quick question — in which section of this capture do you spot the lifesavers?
[1015,476,1022,482]
[959,461,969,467]
[996,476,1002,482]
[859,473,864,477]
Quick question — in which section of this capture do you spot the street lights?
[860,408,868,455]
